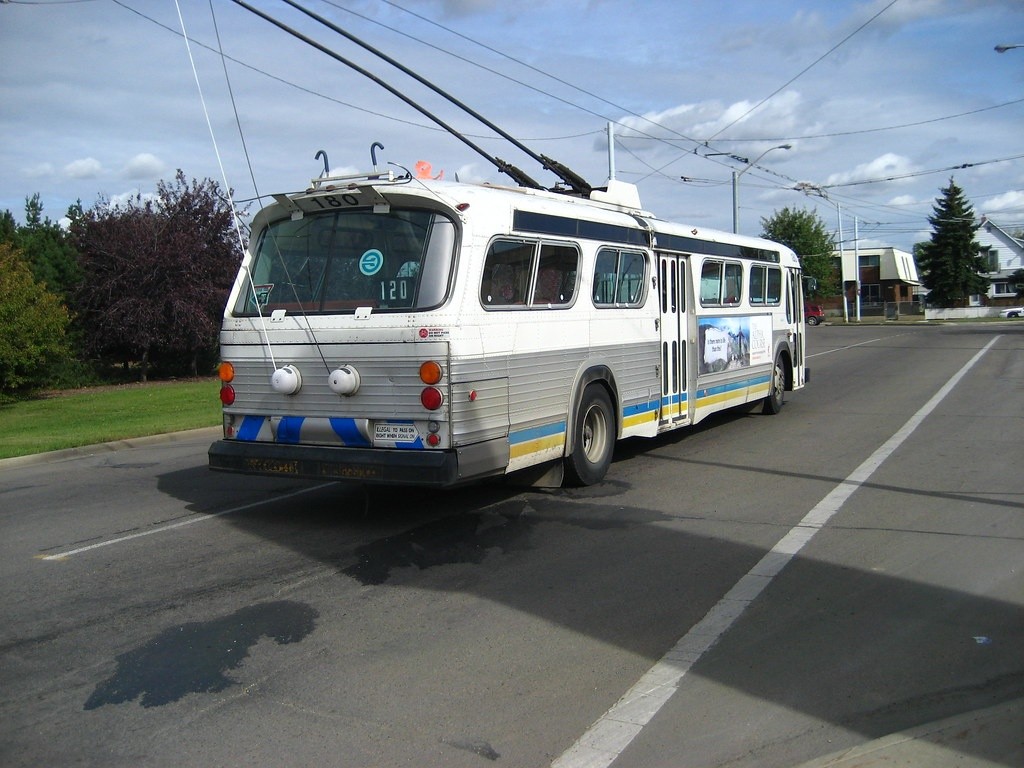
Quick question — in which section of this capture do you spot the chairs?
[701,297,736,303]
[482,263,566,305]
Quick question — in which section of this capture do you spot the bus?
[209,142,817,490]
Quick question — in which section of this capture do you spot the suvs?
[804,303,825,326]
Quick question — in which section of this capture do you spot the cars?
[999,306,1024,318]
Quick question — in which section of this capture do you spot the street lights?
[732,144,792,234]
